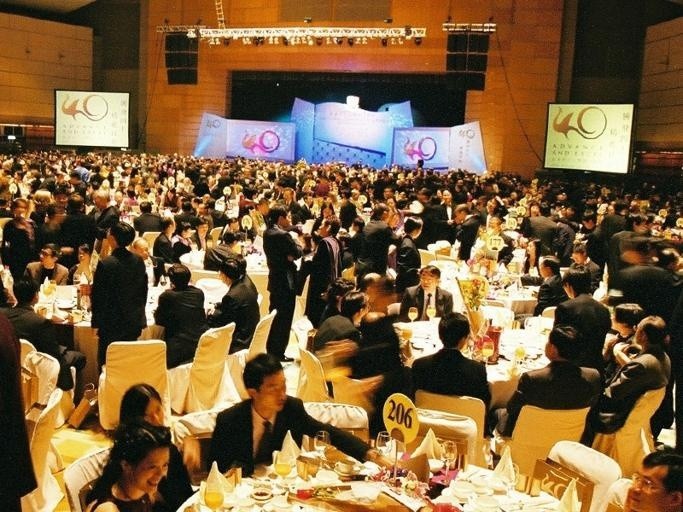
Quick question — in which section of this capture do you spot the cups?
[335,461,353,473]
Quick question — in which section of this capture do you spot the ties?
[425,293,432,320]
[256,421,275,464]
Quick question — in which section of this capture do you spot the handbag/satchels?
[68,388,98,430]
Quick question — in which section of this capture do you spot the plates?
[333,468,354,476]
[247,494,272,504]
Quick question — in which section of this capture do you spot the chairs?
[189,269,219,284]
[207,226,224,250]
[143,231,162,246]
[176,410,224,476]
[298,348,358,404]
[20,339,37,363]
[541,306,558,317]
[167,321,235,414]
[191,277,229,315]
[63,446,110,511]
[97,338,171,430]
[492,405,592,475]
[284,274,312,357]
[24,350,76,409]
[406,407,477,468]
[526,316,553,331]
[331,373,386,424]
[20,388,64,512]
[413,389,493,469]
[305,400,370,447]
[546,438,622,511]
[510,300,539,327]
[592,387,666,478]
[595,478,635,512]
[416,248,457,269]
[342,262,356,281]
[230,308,277,399]
[479,306,516,327]
[486,300,504,308]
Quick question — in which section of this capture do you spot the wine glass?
[408,307,418,325]
[403,327,412,342]
[501,463,519,501]
[441,440,458,484]
[48,279,56,297]
[43,284,51,301]
[313,431,330,471]
[375,431,391,456]
[37,307,47,318]
[513,344,525,377]
[427,305,437,323]
[274,451,291,494]
[79,296,90,316]
[204,485,225,512]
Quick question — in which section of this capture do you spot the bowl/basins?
[476,494,499,512]
[251,488,273,500]
[453,481,475,500]
[427,458,444,473]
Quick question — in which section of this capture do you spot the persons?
[302,160,434,236]
[521,239,548,281]
[656,247,679,283]
[487,326,601,444]
[393,216,425,299]
[400,266,453,322]
[220,218,248,242]
[191,219,211,250]
[203,229,242,272]
[152,217,182,284]
[602,304,644,390]
[548,264,612,444]
[0,308,38,512]
[435,168,683,240]
[304,215,344,328]
[356,203,405,290]
[355,315,412,431]
[90,221,148,377]
[69,243,93,286]
[1,149,94,223]
[172,222,193,257]
[94,154,303,225]
[569,242,601,296]
[18,243,69,307]
[623,448,683,512]
[411,313,498,439]
[673,276,683,453]
[206,352,383,478]
[90,423,171,512]
[205,259,260,356]
[263,205,303,362]
[1,199,40,295]
[128,239,155,285]
[153,264,206,370]
[116,383,194,512]
[313,293,371,354]
[308,279,333,327]
[585,315,672,447]
[531,255,568,317]
[608,240,679,316]
[318,277,357,331]
[8,277,87,391]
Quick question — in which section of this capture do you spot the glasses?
[632,472,665,490]
[39,249,52,256]
